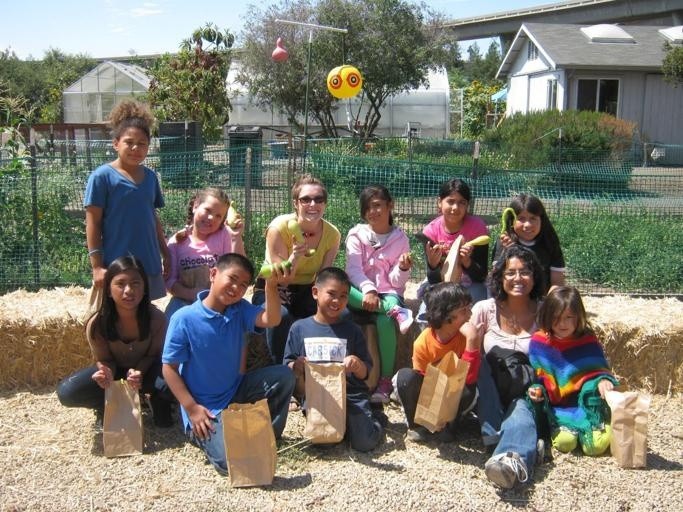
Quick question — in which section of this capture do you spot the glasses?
[295,197,325,204]
[501,270,534,280]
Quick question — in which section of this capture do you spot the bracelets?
[86,249,104,255]
[192,286,198,301]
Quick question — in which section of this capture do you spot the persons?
[281,268,388,453]
[164,189,246,337]
[395,282,485,443]
[85,119,172,306]
[492,195,565,296]
[459,244,555,490]
[252,178,341,365]
[161,254,290,474]
[345,190,415,402]
[418,178,490,320]
[57,258,175,430]
[528,286,615,457]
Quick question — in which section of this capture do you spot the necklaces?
[302,231,314,238]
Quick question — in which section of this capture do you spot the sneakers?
[437,426,457,443]
[95,407,105,424]
[485,452,530,490]
[534,439,547,464]
[148,392,170,427]
[386,303,414,334]
[369,376,391,402]
[405,424,427,440]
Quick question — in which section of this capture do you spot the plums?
[534,389,542,397]
